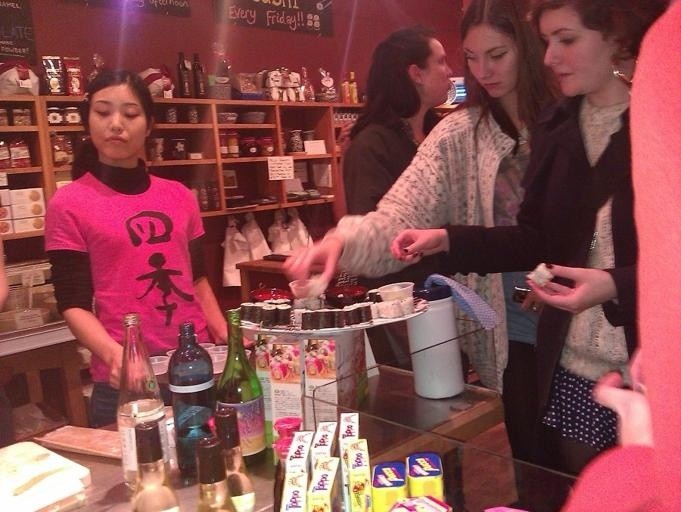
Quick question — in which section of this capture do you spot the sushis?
[240,289,406,330]
[526,263,554,289]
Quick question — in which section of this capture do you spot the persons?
[43,69,230,431]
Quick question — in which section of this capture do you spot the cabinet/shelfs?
[0,93,54,339]
[41,93,225,232]
[276,99,341,209]
[213,100,286,218]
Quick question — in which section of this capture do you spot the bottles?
[342,71,360,106]
[177,49,208,98]
[198,180,221,211]
[129,418,179,512]
[115,312,169,491]
[192,434,236,512]
[214,59,230,86]
[217,307,269,470]
[299,66,315,102]
[269,418,301,511]
[208,409,257,512]
[166,323,216,479]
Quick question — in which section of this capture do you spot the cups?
[172,138,187,160]
[290,278,320,300]
[376,281,415,305]
[150,138,165,161]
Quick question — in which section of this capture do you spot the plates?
[445,78,459,106]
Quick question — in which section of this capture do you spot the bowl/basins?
[217,112,239,124]
[240,111,268,123]
[1,307,50,333]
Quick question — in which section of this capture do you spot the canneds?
[47,107,83,126]
[219,132,274,158]
